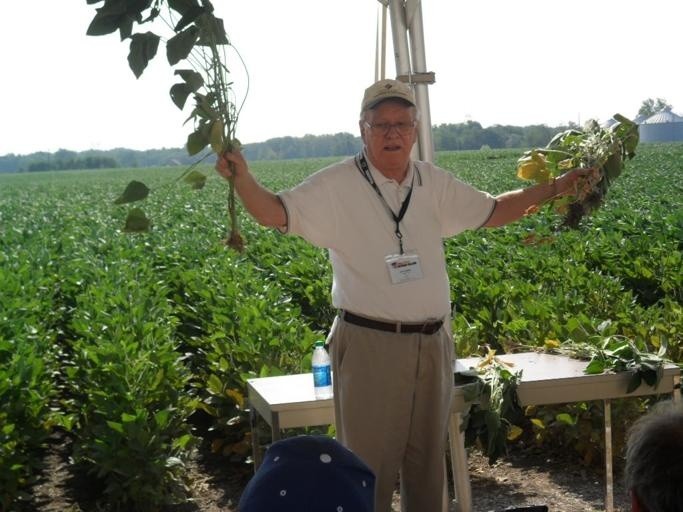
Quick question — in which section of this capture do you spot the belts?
[336,308,446,336]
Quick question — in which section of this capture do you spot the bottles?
[311,340,333,400]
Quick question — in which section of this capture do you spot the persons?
[627,402,683,510]
[217,81,600,511]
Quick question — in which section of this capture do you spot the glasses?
[362,117,420,135]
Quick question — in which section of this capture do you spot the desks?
[246,352,679,512]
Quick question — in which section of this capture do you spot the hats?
[359,79,418,119]
[235,432,378,511]
